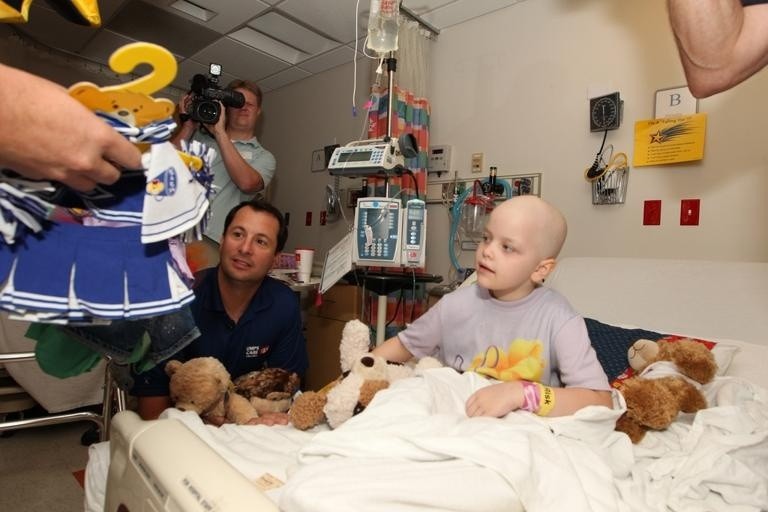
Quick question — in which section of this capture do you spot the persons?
[0,64,143,195]
[369,193,616,418]
[663,1,768,99]
[173,78,280,249]
[130,201,309,427]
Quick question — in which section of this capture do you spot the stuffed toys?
[234,368,301,414]
[615,336,717,444]
[291,316,443,432]
[162,356,258,426]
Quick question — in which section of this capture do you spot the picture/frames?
[652,85,699,120]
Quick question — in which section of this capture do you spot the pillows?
[582,317,741,390]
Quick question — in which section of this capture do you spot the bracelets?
[522,381,539,411]
[540,386,554,416]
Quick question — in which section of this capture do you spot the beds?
[79,314,768,512]
